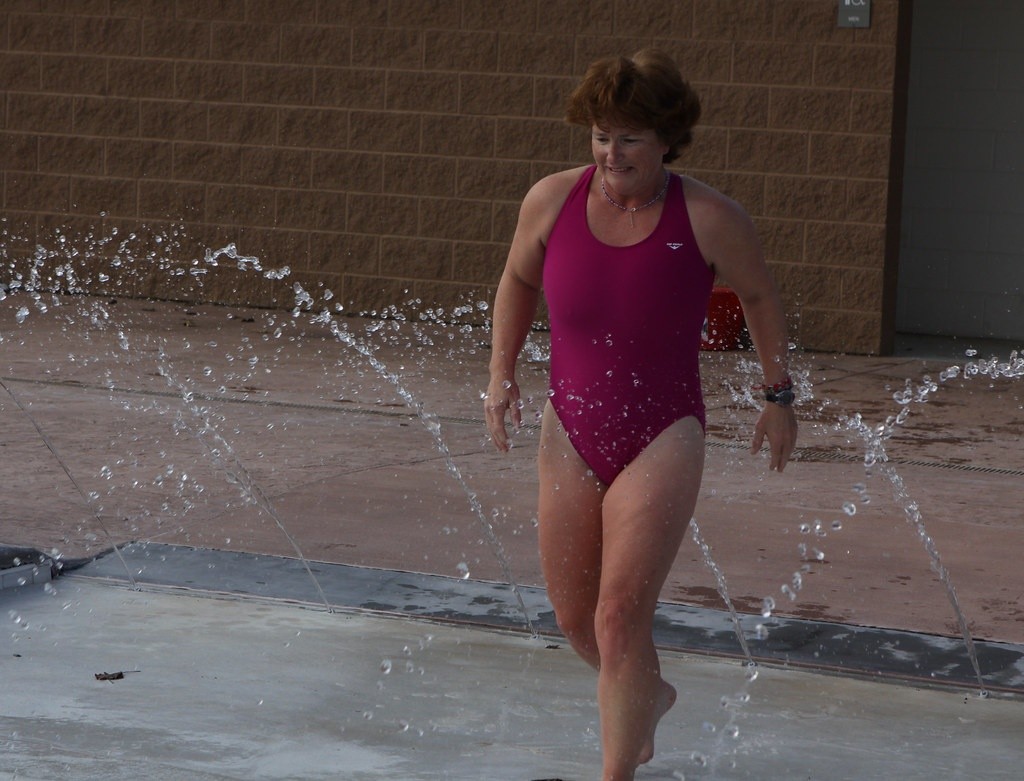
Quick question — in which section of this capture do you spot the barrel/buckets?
[699,286,743,352]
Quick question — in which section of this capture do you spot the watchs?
[765,390,795,406]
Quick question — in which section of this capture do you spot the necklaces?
[601,170,670,212]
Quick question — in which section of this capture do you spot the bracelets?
[751,376,793,393]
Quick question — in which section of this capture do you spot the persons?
[484,48,798,781]
